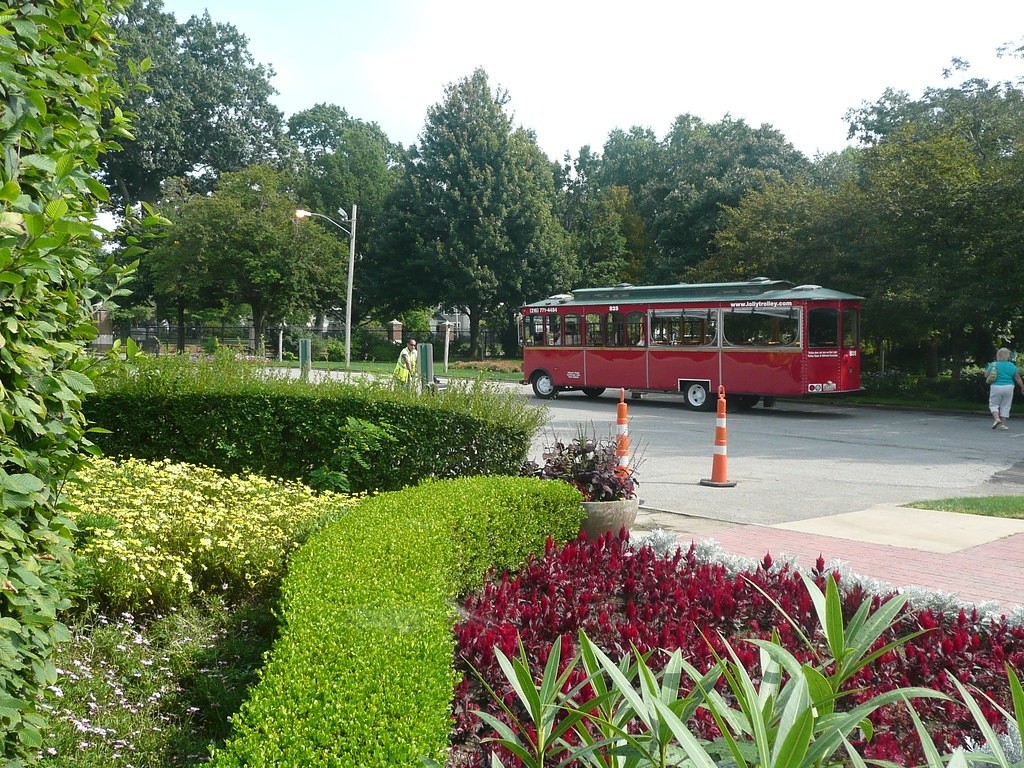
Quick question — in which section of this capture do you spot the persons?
[635,333,644,346]
[986,348,1024,429]
[393,338,417,382]
[552,321,576,345]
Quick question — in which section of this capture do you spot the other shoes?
[1001,425,1008,430]
[992,419,1001,429]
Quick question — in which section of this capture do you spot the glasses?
[409,343,416,347]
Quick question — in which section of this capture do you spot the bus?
[517,276,867,412]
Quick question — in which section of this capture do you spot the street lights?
[295,209,354,366]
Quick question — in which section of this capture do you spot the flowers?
[540,416,650,501]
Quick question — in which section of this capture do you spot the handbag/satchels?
[986,362,996,384]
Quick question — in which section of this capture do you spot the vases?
[577,498,640,543]
